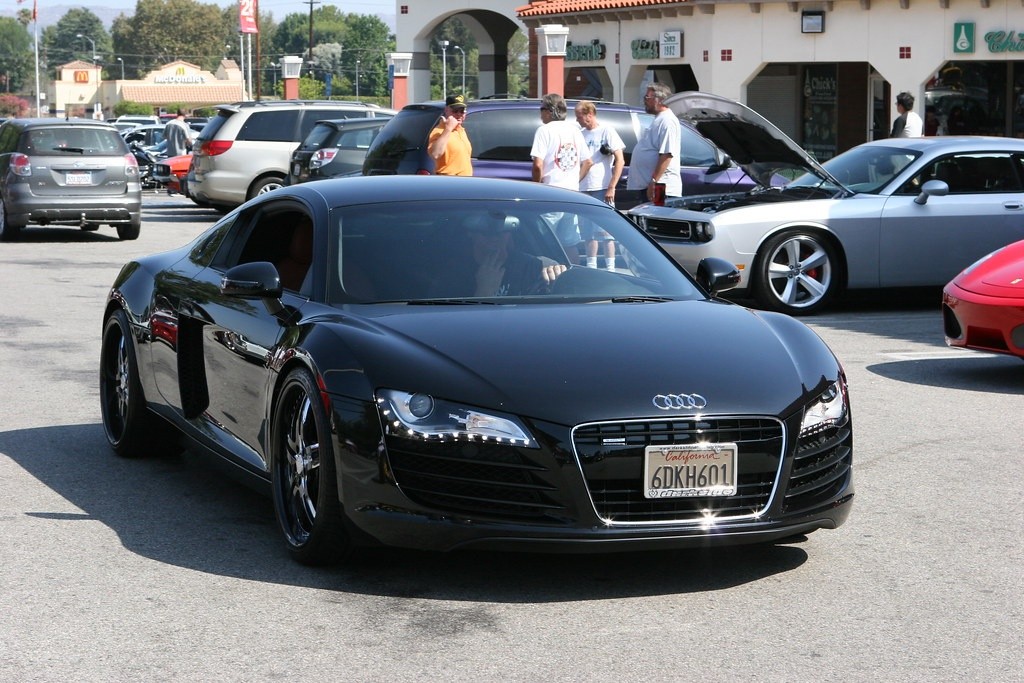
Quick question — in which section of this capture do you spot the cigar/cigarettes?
[494,251,497,255]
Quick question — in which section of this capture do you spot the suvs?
[360,96,796,210]
[184,100,397,214]
[0,115,143,240]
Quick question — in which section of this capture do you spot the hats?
[445,94,467,107]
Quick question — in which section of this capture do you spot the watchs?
[651,178,657,183]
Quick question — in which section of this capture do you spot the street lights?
[355,60,362,102]
[77,34,99,111]
[439,39,452,103]
[235,27,247,101]
[270,62,281,97]
[454,45,466,95]
[117,57,125,80]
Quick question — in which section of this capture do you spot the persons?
[888,92,925,176]
[925,106,940,136]
[162,107,193,197]
[626,83,682,202]
[456,199,567,297]
[530,93,595,265]
[427,95,474,212]
[574,100,626,272]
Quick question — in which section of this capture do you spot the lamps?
[801,10,825,33]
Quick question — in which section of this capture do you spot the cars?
[620,90,1024,314]
[105,114,215,195]
[289,118,392,187]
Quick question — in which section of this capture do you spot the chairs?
[299,236,400,301]
[934,163,960,180]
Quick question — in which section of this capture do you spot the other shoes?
[166,189,179,196]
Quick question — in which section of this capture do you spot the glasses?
[449,108,466,114]
[643,95,654,102]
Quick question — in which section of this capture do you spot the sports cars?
[941,237,1024,364]
[97,180,861,571]
